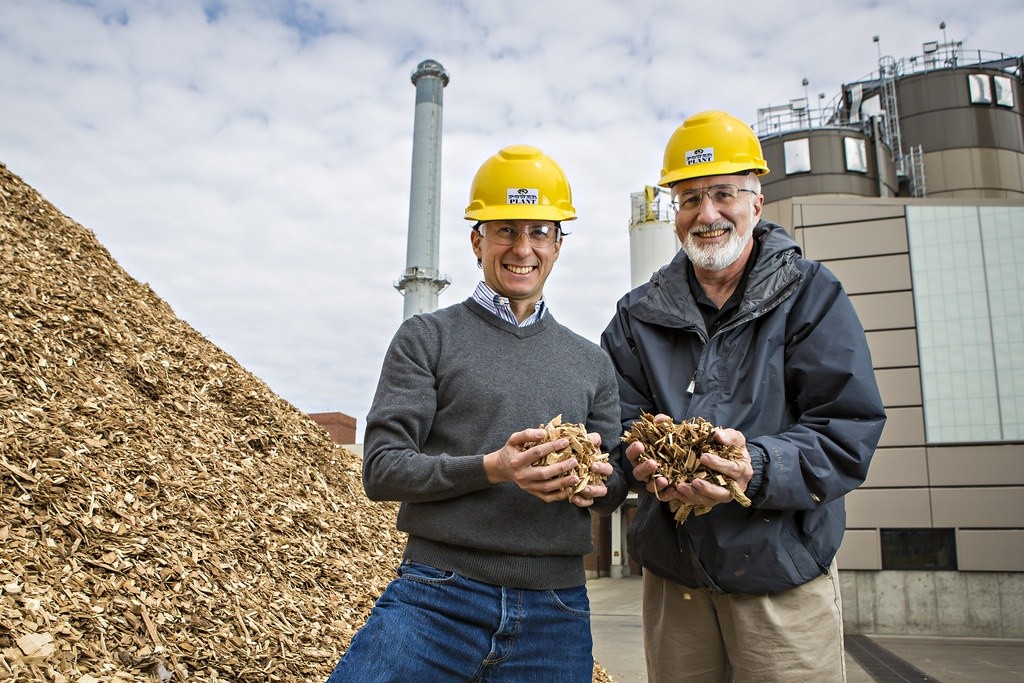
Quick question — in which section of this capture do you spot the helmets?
[464,145,577,222]
[658,110,772,188]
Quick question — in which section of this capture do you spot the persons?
[323,146,627,683]
[602,113,887,683]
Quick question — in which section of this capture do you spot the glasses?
[671,183,758,213]
[472,218,561,249]
